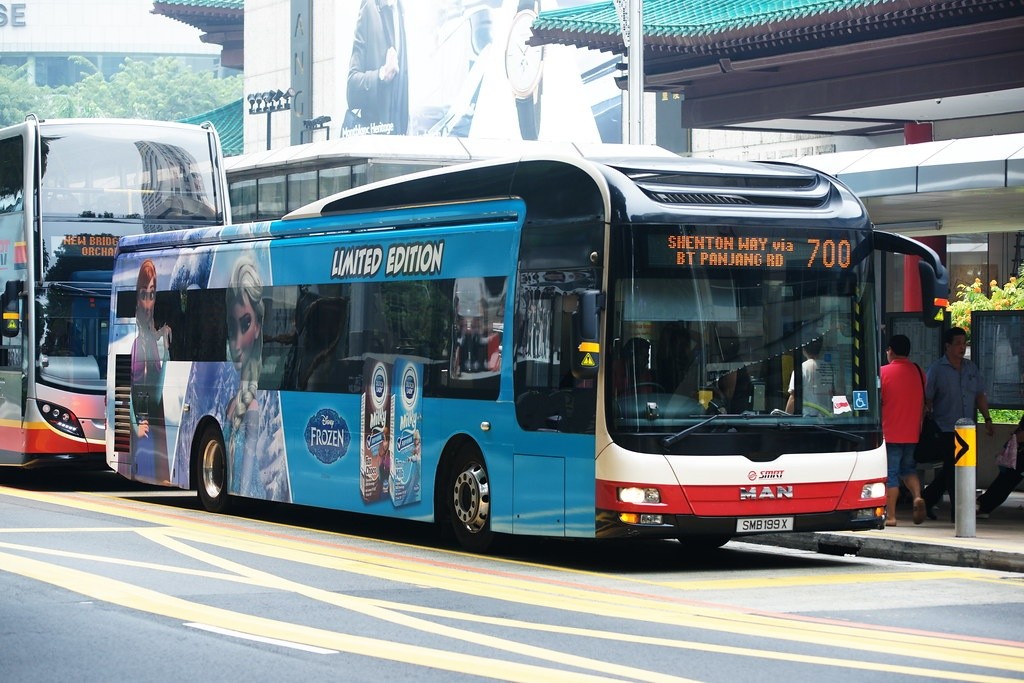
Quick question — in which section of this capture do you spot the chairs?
[38,317,83,349]
[656,326,692,395]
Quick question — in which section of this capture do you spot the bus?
[105,142,950,561]
[45,132,505,227]
[0,112,233,492]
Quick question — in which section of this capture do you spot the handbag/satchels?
[995,434,1017,471]
[921,414,947,464]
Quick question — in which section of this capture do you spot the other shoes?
[927,508,937,520]
[884,516,897,526]
[913,498,926,524]
[977,512,990,519]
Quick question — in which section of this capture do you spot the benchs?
[976,422,1024,494]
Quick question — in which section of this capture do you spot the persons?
[716,327,753,414]
[976,414,1024,519]
[785,333,833,416]
[879,334,926,526]
[613,338,652,398]
[658,322,701,394]
[921,326,995,522]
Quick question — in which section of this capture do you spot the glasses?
[885,348,891,354]
